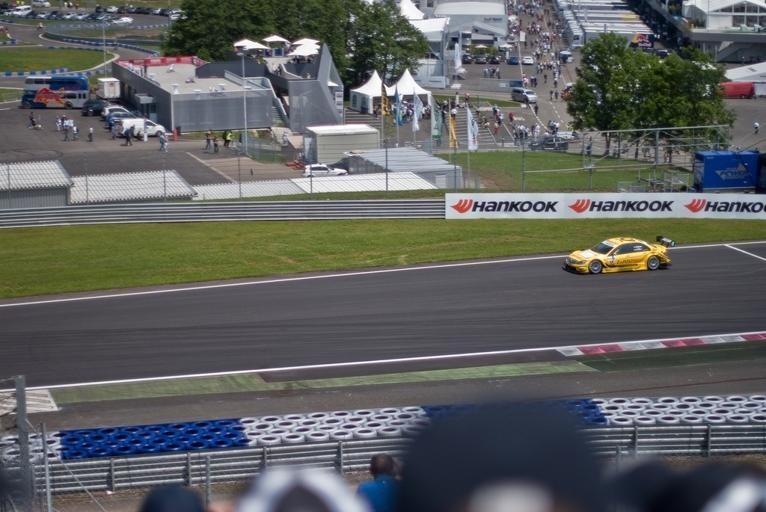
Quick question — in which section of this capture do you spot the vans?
[509,86,538,104]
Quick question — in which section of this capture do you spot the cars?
[558,45,604,106]
[563,234,672,277]
[458,46,537,69]
[80,97,166,140]
[0,0,191,28]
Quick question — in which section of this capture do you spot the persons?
[613,146,617,157]
[753,121,759,134]
[26,112,34,128]
[382,1,575,151]
[663,152,668,162]
[53,111,80,142]
[111,123,135,146]
[87,127,93,142]
[204,127,233,154]
[163,135,168,153]
[160,132,164,150]
[137,401,766,512]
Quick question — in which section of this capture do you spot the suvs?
[301,161,349,179]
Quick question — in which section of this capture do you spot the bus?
[18,73,92,110]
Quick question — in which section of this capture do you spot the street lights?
[234,51,253,159]
